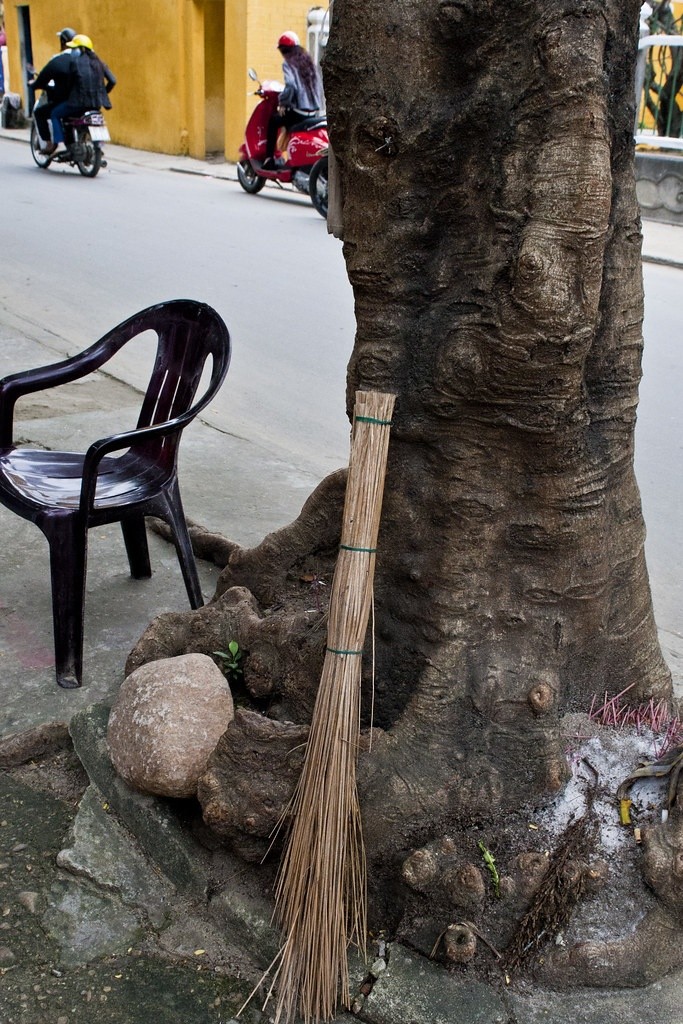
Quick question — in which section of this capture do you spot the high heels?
[260,156,276,169]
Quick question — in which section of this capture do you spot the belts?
[293,107,316,116]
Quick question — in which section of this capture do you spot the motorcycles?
[21,61,107,178]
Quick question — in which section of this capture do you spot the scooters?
[235,67,329,204]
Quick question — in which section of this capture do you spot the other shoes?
[39,143,57,155]
[49,146,67,158]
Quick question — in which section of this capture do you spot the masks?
[71,48,81,56]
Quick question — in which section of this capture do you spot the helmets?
[56,28,76,41]
[277,31,300,48]
[66,34,93,50]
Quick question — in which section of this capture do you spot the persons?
[29,27,117,159]
[261,32,320,170]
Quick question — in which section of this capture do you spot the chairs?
[0,297,232,689]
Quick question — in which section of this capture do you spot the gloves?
[277,104,290,118]
